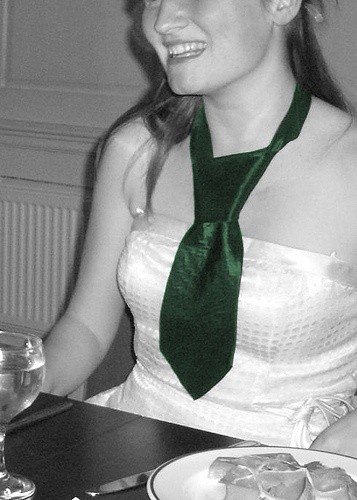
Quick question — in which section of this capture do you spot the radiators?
[0,176,92,351]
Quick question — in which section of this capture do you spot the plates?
[147,446,356,500]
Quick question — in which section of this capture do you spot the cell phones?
[6,392,74,435]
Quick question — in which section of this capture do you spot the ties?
[157,82,313,401]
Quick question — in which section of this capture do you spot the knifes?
[84,440,262,497]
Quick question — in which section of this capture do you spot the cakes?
[207,452,356,500]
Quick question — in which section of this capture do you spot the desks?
[0,392,266,500]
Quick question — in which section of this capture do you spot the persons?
[39,1,357,461]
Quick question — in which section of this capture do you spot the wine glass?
[0,330,47,499]
